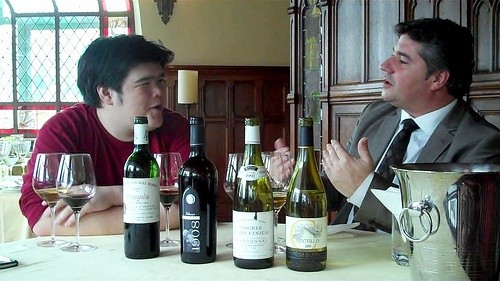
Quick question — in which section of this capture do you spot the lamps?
[153,0,177,25]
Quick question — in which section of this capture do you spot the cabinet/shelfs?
[165,66,289,222]
[289,1,332,178]
[470,0,500,129]
[412,0,469,25]
[332,103,367,150]
[340,0,406,84]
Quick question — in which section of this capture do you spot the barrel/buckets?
[389,163,500,281]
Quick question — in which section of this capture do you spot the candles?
[177,70,199,105]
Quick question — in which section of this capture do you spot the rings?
[328,164,333,169]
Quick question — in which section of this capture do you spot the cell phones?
[0,254,18,268]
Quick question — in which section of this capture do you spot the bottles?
[286,118,327,272]
[122,116,160,260]
[177,118,217,265]
[232,119,274,269]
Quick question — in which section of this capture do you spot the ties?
[351,120,420,233]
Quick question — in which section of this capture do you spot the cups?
[391,214,410,266]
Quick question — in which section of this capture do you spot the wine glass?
[223,153,245,249]
[0,134,32,190]
[31,153,68,248]
[152,153,184,247]
[259,153,294,255]
[55,155,98,252]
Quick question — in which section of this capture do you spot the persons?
[18,33,234,239]
[269,18,500,235]
